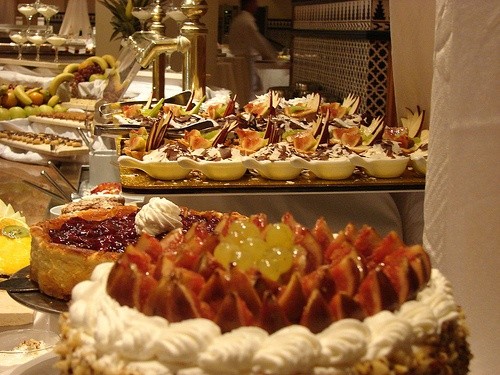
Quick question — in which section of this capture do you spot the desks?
[256,60,290,89]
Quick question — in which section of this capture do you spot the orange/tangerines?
[0,90,43,107]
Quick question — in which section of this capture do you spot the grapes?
[71,72,86,82]
[81,65,103,81]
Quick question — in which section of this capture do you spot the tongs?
[94,120,220,139]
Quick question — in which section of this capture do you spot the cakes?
[29,182,476,375]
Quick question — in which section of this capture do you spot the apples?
[0,105,67,122]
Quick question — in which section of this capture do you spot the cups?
[89,150,121,188]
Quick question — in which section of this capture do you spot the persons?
[224,0,290,131]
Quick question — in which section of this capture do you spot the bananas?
[14,53,116,105]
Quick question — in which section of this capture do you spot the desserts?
[36,111,87,121]
[0,129,83,147]
[114,93,430,182]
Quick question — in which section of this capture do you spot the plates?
[73,194,116,203]
[49,204,78,216]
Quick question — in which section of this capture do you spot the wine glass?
[167,7,186,36]
[27,31,47,61]
[9,29,28,60]
[35,3,60,36]
[45,31,68,63]
[18,3,38,36]
[131,6,154,31]
